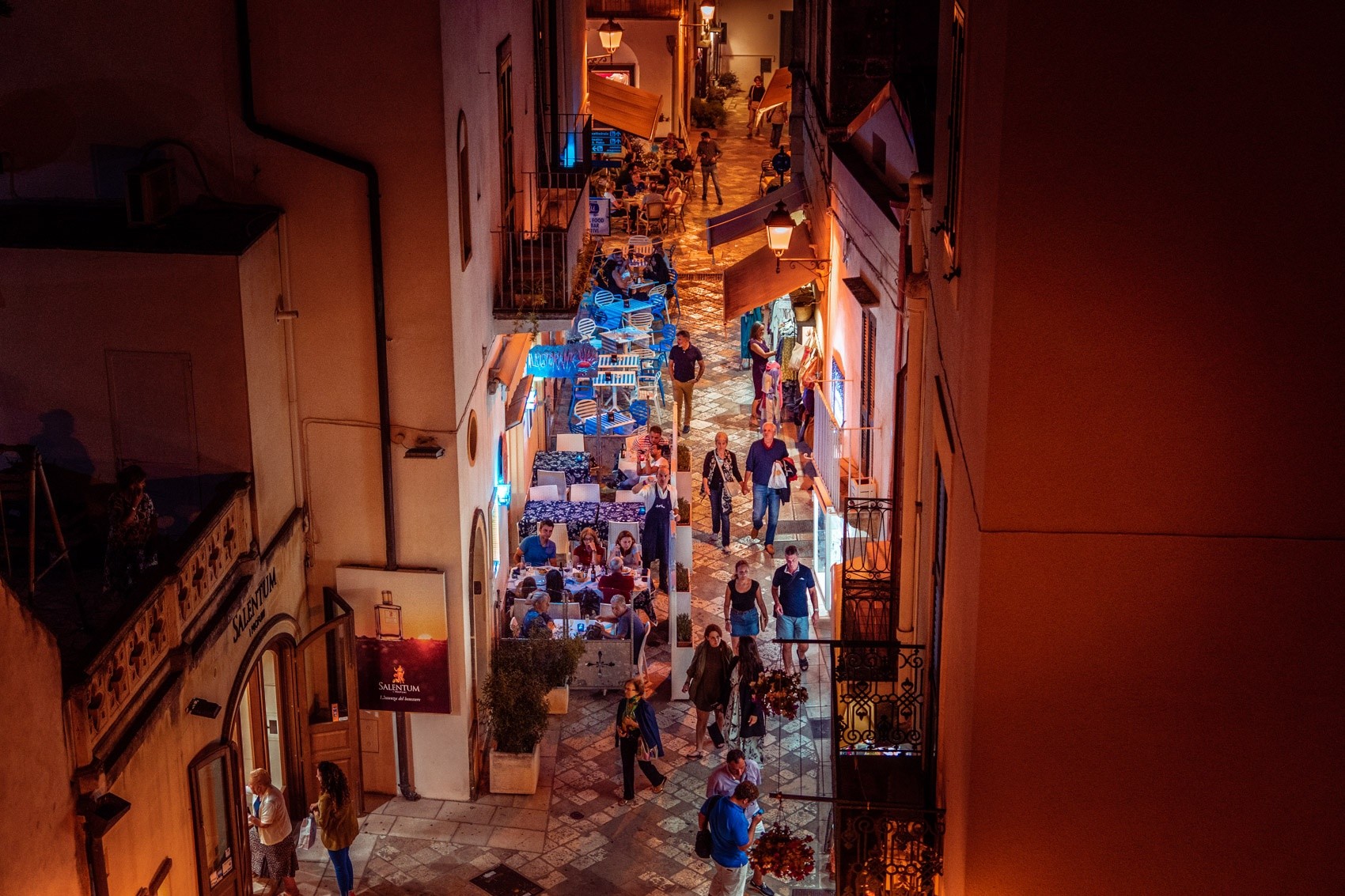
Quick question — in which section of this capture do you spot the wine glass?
[585,614,590,626]
[564,564,570,579]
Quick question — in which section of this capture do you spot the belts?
[732,606,756,614]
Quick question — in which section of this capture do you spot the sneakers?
[747,878,774,896]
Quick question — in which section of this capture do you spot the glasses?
[649,447,657,450]
[583,537,594,541]
[748,800,755,806]
[624,687,636,692]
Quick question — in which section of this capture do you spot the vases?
[766,698,792,715]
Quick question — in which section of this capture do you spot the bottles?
[621,442,625,458]
[374,589,403,641]
[604,559,609,575]
[642,564,647,582]
[637,561,642,576]
[519,555,526,578]
[558,561,564,579]
[591,564,596,582]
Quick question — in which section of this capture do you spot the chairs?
[507,147,716,698]
[759,150,805,207]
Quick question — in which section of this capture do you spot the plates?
[621,566,632,574]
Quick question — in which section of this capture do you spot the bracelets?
[696,378,699,382]
[725,618,731,623]
[642,479,649,486]
[684,681,690,685]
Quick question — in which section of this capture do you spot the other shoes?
[618,798,634,806]
[723,545,732,553]
[686,750,703,759]
[765,544,775,554]
[652,776,667,793]
[683,425,690,433]
[658,582,668,594]
[751,527,759,539]
[712,533,718,540]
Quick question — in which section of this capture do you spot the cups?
[526,562,531,572]
[545,562,551,571]
[576,562,583,572]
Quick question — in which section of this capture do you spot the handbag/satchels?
[767,462,788,489]
[724,481,739,497]
[694,828,712,859]
[297,810,317,850]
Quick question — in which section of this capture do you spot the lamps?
[586,13,627,74]
[763,198,833,281]
[677,1,716,36]
[187,697,225,719]
[403,436,446,462]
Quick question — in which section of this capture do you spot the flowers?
[744,822,819,886]
[749,665,811,721]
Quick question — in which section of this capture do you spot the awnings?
[752,65,792,130]
[721,219,821,326]
[702,178,809,253]
[587,70,665,141]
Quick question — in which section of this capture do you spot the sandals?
[797,649,809,671]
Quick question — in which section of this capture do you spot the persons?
[697,130,725,205]
[717,633,766,771]
[605,530,642,568]
[632,426,670,459]
[702,431,751,554]
[571,527,605,567]
[614,677,667,807]
[695,782,763,896]
[747,320,779,428]
[594,132,693,234]
[597,556,636,605]
[667,330,705,435]
[721,559,769,656]
[739,419,791,554]
[745,75,766,137]
[512,519,558,570]
[515,590,557,639]
[593,594,645,699]
[520,578,541,597]
[771,545,820,676]
[765,101,788,149]
[704,747,777,896]
[682,622,735,760]
[544,568,576,603]
[245,767,302,896]
[612,443,669,492]
[309,760,359,896]
[632,466,681,595]
[596,235,674,302]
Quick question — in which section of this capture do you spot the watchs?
[813,607,819,611]
[247,814,252,821]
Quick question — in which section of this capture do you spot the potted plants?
[503,615,585,715]
[471,665,552,796]
